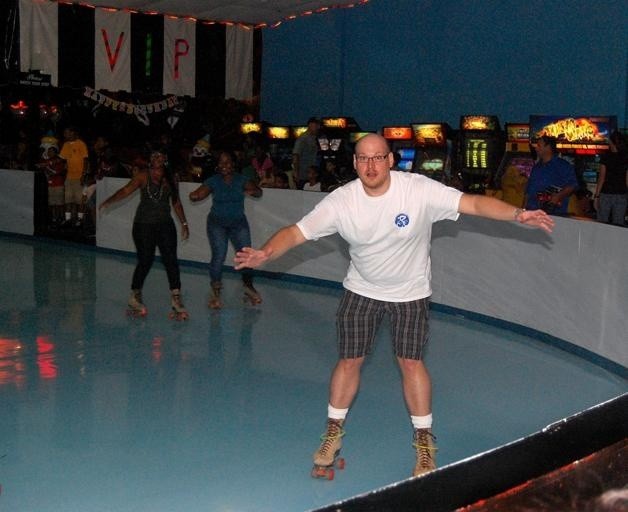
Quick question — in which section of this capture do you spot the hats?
[307,116,325,124]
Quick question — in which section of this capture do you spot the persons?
[189,147,263,304]
[229,132,556,476]
[97,142,189,316]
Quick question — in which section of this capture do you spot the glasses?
[354,153,389,165]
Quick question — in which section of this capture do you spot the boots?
[411,427,440,481]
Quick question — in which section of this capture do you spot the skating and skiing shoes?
[206,280,225,312]
[123,287,149,321]
[167,287,191,322]
[237,278,264,308]
[309,416,347,481]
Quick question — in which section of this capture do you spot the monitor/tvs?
[463,138,489,168]
[582,170,599,183]
[506,155,534,178]
[561,154,576,167]
[395,148,418,171]
[418,157,447,172]
